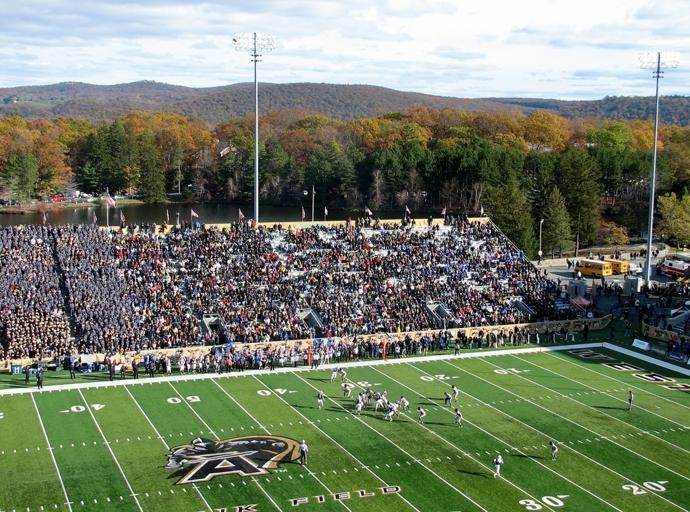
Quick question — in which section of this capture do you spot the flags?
[40,205,484,224]
[105,192,119,210]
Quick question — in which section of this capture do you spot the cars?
[0,188,99,208]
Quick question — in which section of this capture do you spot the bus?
[604,258,627,275]
[576,260,613,279]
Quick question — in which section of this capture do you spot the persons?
[589,248,689,367]
[257,211,590,371]
[316,371,464,428]
[492,455,504,478]
[628,390,633,410]
[298,439,308,465]
[548,441,558,461]
[0,218,258,390]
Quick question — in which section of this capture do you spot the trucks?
[655,256,690,282]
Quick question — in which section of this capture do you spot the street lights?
[636,50,679,289]
[539,219,545,261]
[232,30,279,229]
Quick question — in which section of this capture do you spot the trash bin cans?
[12,365,22,375]
